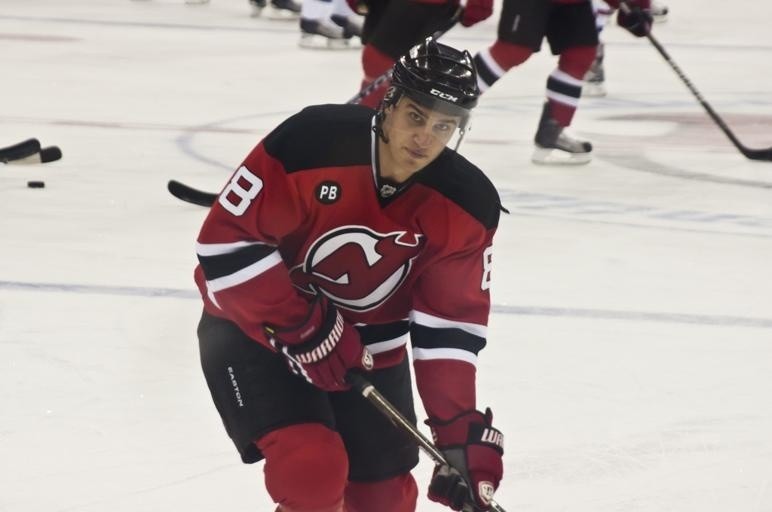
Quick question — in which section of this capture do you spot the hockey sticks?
[168,8,465,209]
[614,0,772,163]
[0,138,63,167]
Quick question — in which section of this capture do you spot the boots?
[583,59,606,83]
[534,102,592,153]
[299,1,366,38]
[248,0,302,12]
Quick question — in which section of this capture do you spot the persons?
[194,38,505,510]
[250,0,655,155]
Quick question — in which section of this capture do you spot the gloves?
[617,6,654,36]
[460,0,494,27]
[424,407,504,512]
[264,295,374,392]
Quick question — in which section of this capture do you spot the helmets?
[381,36,481,109]
